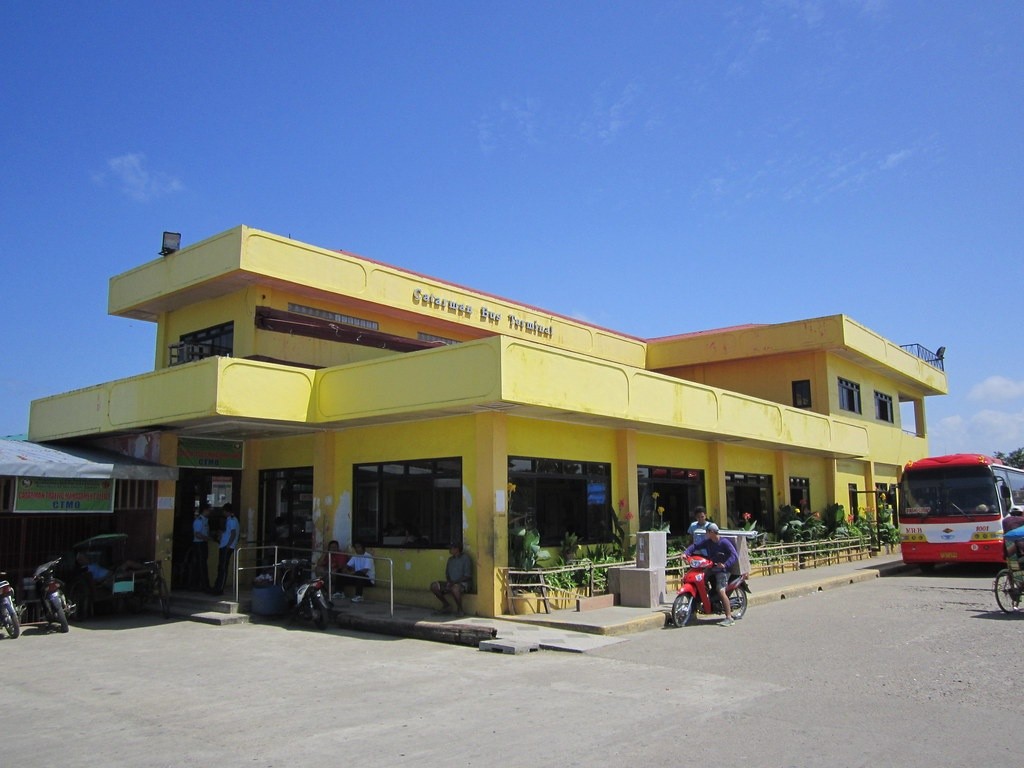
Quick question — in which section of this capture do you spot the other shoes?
[432,610,449,616]
[457,611,465,617]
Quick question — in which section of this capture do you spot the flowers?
[507,478,898,590]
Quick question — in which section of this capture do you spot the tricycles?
[993,525,1024,614]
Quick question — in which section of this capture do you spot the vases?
[506,542,899,616]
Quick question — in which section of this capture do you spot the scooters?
[274,558,334,632]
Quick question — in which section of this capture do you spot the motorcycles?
[671,544,752,628]
[31,555,77,633]
[0,571,27,638]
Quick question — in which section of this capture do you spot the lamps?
[936,346,945,357]
[156,230,183,256]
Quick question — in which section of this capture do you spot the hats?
[703,523,719,531]
[1010,505,1023,512]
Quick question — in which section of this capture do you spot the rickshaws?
[72,532,172,621]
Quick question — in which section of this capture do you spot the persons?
[332,542,375,601]
[430,539,472,618]
[681,523,738,627]
[685,506,719,555]
[193,506,211,593]
[208,503,240,596]
[1002,506,1024,600]
[315,540,345,578]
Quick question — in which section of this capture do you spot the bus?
[885,453,1024,574]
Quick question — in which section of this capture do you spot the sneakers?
[720,618,735,626]
[351,595,363,602]
[332,592,345,599]
